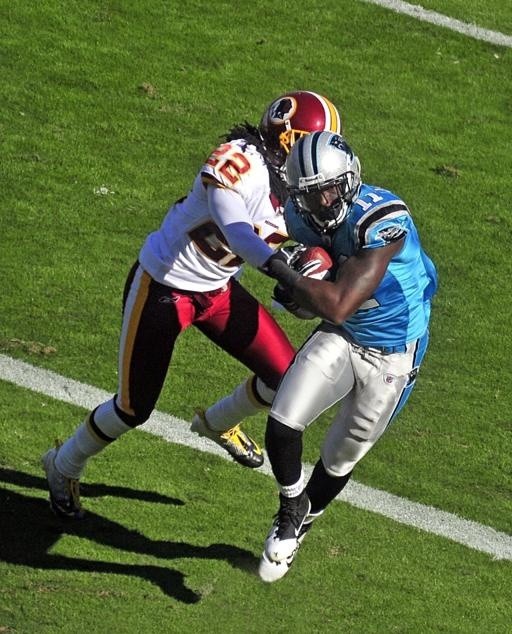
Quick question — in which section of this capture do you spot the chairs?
[41,446,91,522]
[257,491,324,583]
[190,407,264,467]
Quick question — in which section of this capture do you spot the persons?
[43,89,344,518]
[256,131,442,581]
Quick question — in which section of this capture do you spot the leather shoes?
[268,242,324,313]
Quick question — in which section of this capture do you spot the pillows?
[260,90,341,163]
[285,131,361,236]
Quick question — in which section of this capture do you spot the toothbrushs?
[289,246,333,320]
